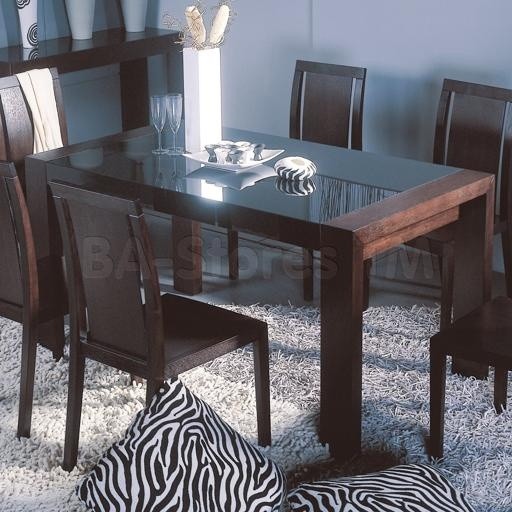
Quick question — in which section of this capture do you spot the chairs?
[0,159,139,441]
[403,77,511,299]
[46,179,275,470]
[288,59,368,152]
[427,244,512,461]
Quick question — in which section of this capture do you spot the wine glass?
[204,141,266,165]
[150,95,170,155]
[166,92,185,156]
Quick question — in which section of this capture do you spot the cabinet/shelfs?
[184,48,222,154]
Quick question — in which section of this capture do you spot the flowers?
[162,0,237,49]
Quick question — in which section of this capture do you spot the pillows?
[286,456,473,511]
[82,377,287,512]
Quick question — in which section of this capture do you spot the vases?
[16,0,148,49]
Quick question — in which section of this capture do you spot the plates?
[180,144,286,174]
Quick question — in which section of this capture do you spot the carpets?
[0,305,512,512]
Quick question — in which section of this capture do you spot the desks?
[0,26,184,133]
[30,115,498,456]
